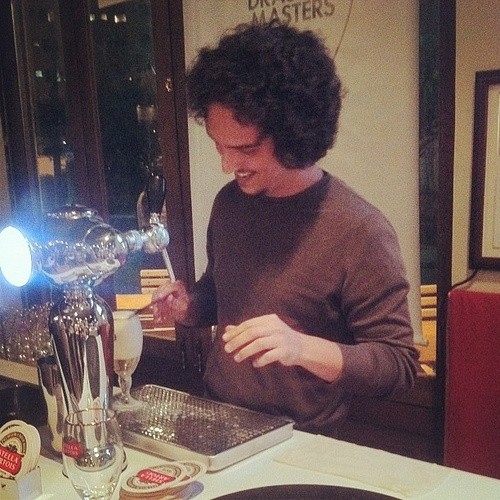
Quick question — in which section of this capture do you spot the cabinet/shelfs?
[0,0,194,291]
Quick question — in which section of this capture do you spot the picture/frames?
[469,69,500,270]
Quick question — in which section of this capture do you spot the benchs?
[117,269,437,363]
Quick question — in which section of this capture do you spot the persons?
[149,19,419,438]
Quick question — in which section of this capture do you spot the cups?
[62,408,121,500]
[36,355,67,456]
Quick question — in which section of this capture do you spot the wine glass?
[110,312,144,411]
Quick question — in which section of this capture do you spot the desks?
[1,384,499,499]
